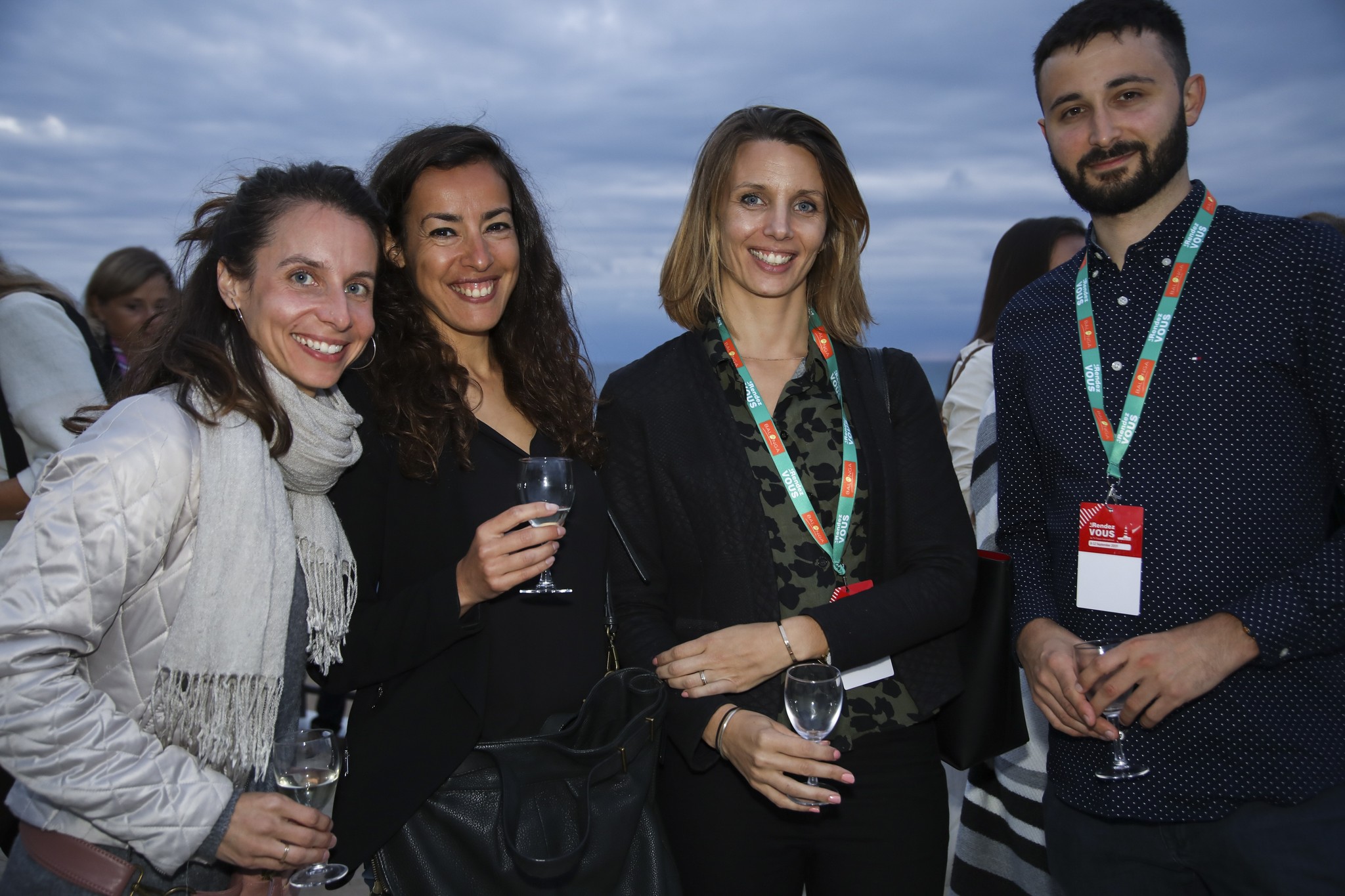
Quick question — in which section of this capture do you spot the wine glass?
[1075,638,1150,781]
[271,730,350,887]
[785,661,843,809]
[512,454,581,599]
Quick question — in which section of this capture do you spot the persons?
[311,120,675,896]
[0,158,386,896]
[81,244,182,404]
[0,251,120,551]
[940,218,1090,895]
[994,0,1344,894]
[590,105,977,895]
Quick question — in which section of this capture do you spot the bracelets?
[774,619,801,667]
[716,706,745,759]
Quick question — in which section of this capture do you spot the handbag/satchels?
[369,668,684,895]
[190,867,306,896]
[932,549,1030,770]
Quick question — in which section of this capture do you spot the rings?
[700,670,707,686]
[279,843,291,864]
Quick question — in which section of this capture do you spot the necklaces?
[741,356,807,361]
